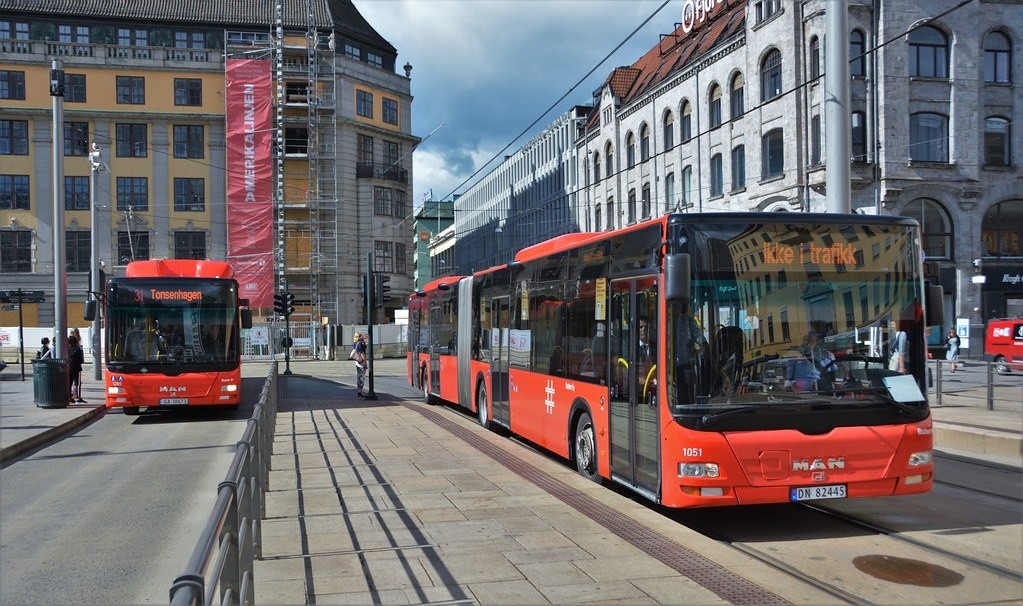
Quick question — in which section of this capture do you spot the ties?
[642,345,647,358]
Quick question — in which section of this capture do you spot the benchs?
[0,346,42,377]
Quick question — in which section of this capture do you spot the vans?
[984,319,1023,376]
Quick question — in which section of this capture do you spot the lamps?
[403,61,413,77]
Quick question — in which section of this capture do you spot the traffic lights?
[376,274,391,308]
[284,294,295,316]
[274,293,283,314]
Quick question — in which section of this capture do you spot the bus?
[84,259,253,415]
[406,211,945,509]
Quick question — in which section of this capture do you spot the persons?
[350,332,368,398]
[472,319,482,359]
[155,324,225,354]
[888,321,906,374]
[614,316,656,395]
[946,328,960,373]
[674,303,839,404]
[40,327,88,404]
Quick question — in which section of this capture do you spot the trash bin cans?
[30,358,68,409]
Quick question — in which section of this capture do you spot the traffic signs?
[0,290,46,311]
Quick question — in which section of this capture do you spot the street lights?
[89,152,112,380]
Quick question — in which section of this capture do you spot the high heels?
[358,392,365,399]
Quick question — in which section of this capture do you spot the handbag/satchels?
[944,343,950,349]
[350,341,366,362]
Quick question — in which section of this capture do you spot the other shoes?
[951,369,955,373]
[76,398,88,404]
[69,398,76,403]
